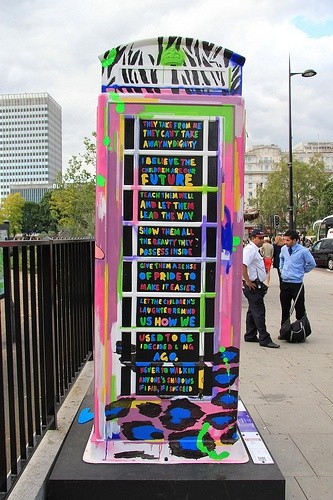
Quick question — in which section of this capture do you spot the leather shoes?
[246,338,280,347]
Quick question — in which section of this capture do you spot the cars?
[308,239,333,271]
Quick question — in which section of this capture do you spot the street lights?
[289,51,318,231]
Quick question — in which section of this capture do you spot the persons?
[276,230,317,341]
[242,229,281,349]
[243,230,316,252]
[260,234,274,288]
[272,235,283,288]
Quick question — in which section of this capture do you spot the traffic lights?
[274,214,280,229]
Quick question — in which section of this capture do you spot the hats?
[250,229,268,238]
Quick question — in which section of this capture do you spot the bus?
[312,215,333,242]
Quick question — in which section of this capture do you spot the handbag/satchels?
[279,314,311,343]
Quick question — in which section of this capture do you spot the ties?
[288,246,293,255]
